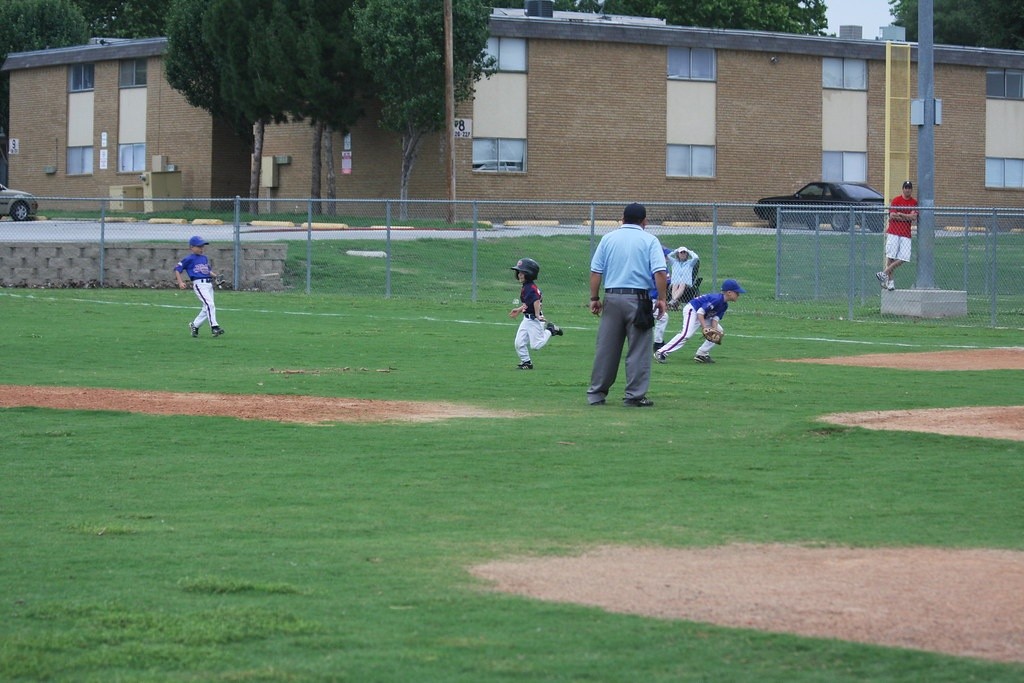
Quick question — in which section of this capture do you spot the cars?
[0,184,41,220]
[752,182,885,232]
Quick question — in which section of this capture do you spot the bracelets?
[591,297,599,301]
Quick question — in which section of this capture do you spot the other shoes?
[653,341,665,352]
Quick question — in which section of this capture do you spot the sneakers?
[887,280,896,290]
[517,360,533,369]
[876,272,889,289]
[623,395,654,406]
[212,328,224,337]
[189,322,199,337]
[653,351,665,363]
[548,322,563,336]
[591,399,606,405]
[693,353,715,364]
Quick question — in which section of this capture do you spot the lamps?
[96,38,111,46]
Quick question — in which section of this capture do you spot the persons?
[654,279,746,364]
[649,268,671,352]
[876,181,918,291]
[587,204,667,407]
[174,235,225,337]
[508,258,563,370]
[667,247,699,308]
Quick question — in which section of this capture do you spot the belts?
[202,279,211,283]
[525,311,542,319]
[604,288,648,294]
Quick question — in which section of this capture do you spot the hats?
[677,246,688,253]
[189,236,209,247]
[903,181,913,188]
[721,279,748,294]
[624,202,646,225]
[662,246,672,255]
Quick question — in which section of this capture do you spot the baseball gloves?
[214,274,225,285]
[702,326,723,345]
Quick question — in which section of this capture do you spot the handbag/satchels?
[632,294,655,329]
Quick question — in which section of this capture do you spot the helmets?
[511,258,539,280]
